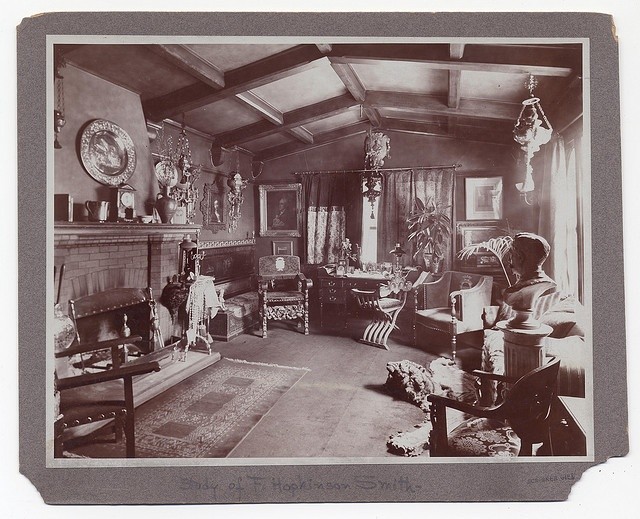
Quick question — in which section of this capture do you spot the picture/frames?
[456,222,506,254]
[201,181,226,235]
[258,182,302,237]
[273,240,294,254]
[465,176,504,220]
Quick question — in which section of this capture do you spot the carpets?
[63,356,311,460]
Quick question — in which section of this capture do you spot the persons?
[269,196,293,230]
[211,200,222,222]
[500,232,560,311]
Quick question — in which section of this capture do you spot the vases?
[155,186,178,224]
[159,126,189,185]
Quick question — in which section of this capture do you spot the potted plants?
[406,198,450,275]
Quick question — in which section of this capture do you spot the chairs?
[54,336,160,459]
[413,271,493,361]
[259,255,313,339]
[352,289,407,351]
[428,356,561,462]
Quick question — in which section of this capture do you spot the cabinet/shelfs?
[319,276,347,328]
